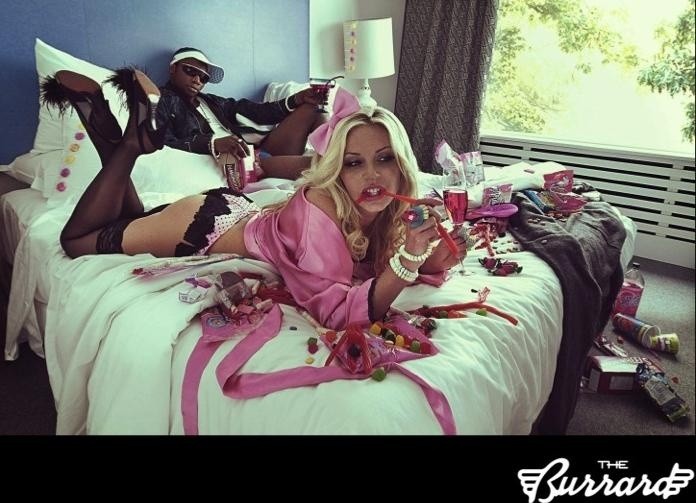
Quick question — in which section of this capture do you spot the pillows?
[0,37,228,212]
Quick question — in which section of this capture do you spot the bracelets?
[284,96,296,113]
[389,241,433,282]
[211,135,221,162]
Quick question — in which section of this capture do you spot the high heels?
[40,69,124,147]
[104,65,177,155]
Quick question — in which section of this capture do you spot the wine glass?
[310,78,334,114]
[442,164,472,271]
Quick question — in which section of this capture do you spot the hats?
[169,50,225,84]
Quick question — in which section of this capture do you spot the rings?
[236,137,243,143]
[400,204,430,228]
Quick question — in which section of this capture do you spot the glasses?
[178,62,211,84]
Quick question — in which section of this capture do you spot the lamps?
[344,16,396,109]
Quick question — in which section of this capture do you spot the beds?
[1,159,638,435]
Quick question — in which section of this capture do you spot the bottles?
[614,262,645,319]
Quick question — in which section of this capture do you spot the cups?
[612,313,660,348]
[648,333,680,354]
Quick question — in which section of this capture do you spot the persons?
[41,62,443,333]
[155,47,331,182]
